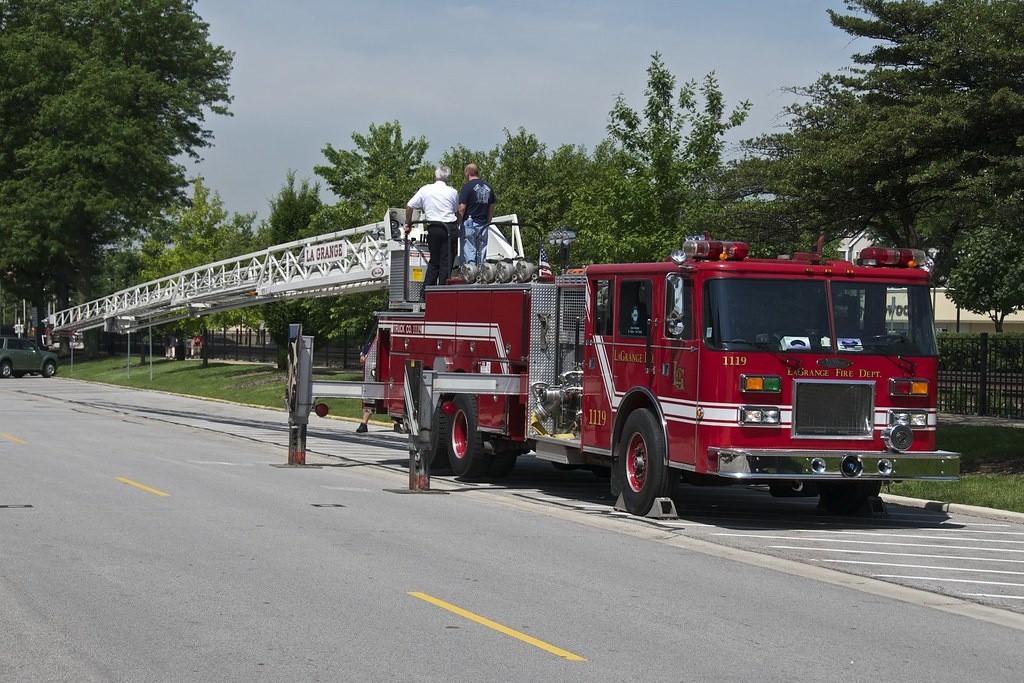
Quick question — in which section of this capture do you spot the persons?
[456,164,495,265]
[165,336,202,360]
[404,165,459,301]
[356,342,403,433]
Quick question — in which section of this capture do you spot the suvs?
[0,335,58,378]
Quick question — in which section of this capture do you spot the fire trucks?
[42,208,959,517]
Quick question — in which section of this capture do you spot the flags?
[540,247,553,279]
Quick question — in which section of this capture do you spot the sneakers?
[356,423,368,432]
[394,424,403,432]
